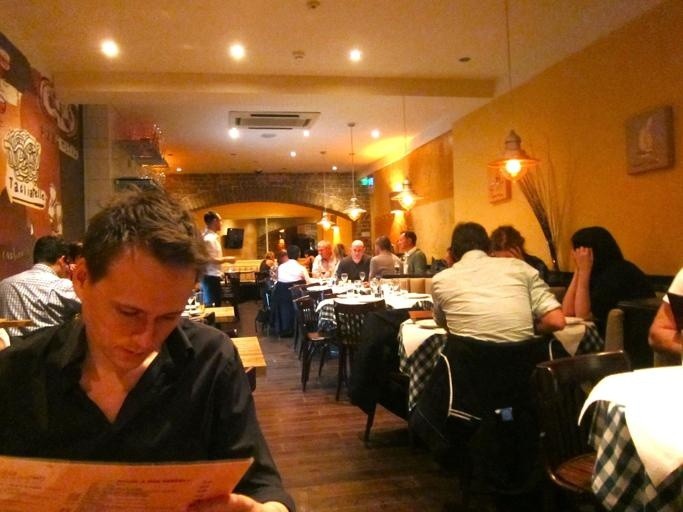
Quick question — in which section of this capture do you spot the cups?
[193,292,205,315]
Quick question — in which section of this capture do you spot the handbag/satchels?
[256,309,270,323]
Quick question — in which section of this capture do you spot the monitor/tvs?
[225,228,243,248]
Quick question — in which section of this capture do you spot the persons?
[430,221,658,342]
[257,231,427,341]
[649,263,682,356]
[0,182,292,511]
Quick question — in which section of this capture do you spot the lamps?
[316,152,335,228]
[390,95,423,210]
[490,0,542,182]
[342,121,367,222]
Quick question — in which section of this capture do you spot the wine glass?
[338,272,400,297]
[186,291,194,315]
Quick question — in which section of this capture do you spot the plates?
[563,316,585,325]
[415,318,439,328]
